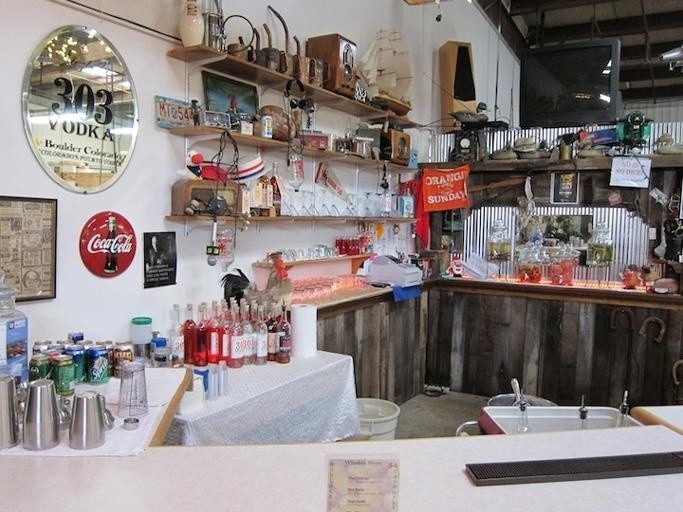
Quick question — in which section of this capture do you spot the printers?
[369,255,423,287]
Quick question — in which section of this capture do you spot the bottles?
[585,221,616,266]
[166,294,292,368]
[240,160,284,218]
[261,112,273,140]
[191,350,229,402]
[130,314,168,368]
[288,138,305,192]
[0,287,31,425]
[515,240,581,288]
[103,215,118,275]
[336,236,368,257]
[487,218,512,262]
[178,0,205,48]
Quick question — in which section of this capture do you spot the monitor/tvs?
[520,37,621,128]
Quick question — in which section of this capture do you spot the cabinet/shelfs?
[163,44,424,239]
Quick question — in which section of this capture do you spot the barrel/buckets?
[357,398,400,439]
[456,420,480,435]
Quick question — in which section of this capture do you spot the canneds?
[29,332,134,396]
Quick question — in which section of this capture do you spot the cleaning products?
[381,182,392,218]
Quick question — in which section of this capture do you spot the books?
[323,451,403,512]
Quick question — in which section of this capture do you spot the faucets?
[579,392,589,430]
[509,377,531,433]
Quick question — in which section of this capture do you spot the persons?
[444,255,464,279]
[145,235,170,275]
[187,99,203,127]
[617,263,644,291]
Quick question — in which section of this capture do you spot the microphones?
[207,218,219,266]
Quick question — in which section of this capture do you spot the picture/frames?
[200,70,259,117]
[0,194,58,303]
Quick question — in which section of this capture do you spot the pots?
[487,392,560,408]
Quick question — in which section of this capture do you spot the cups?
[115,361,149,420]
[67,390,107,451]
[290,275,372,303]
[20,379,62,451]
[0,374,18,450]
[280,191,386,216]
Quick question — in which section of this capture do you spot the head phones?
[381,165,388,189]
[185,197,208,215]
[283,78,307,109]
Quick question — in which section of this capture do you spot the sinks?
[480,404,643,435]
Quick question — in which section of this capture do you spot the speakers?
[357,129,411,166]
[308,35,357,98]
[172,180,242,216]
[439,41,477,132]
[455,132,477,161]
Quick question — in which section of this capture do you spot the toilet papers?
[291,305,317,358]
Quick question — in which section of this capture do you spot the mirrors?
[19,23,141,197]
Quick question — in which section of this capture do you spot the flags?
[420,163,471,212]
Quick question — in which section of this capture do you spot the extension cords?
[425,384,449,393]
